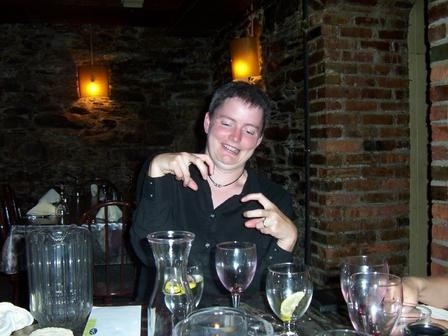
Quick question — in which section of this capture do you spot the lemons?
[165,281,196,293]
[280,291,306,321]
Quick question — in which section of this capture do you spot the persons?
[383,275,448,329]
[131,81,299,304]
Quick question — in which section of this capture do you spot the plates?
[81,306,141,336]
[28,327,74,336]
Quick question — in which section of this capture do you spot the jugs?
[27,226,95,332]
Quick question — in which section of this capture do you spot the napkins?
[82,305,141,336]
[26,199,56,216]
[39,189,61,202]
[0,302,34,336]
[96,204,123,222]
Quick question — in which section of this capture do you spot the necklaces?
[209,169,244,187]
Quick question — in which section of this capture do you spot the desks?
[0,223,137,310]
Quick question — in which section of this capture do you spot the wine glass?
[266,262,313,336]
[215,241,256,308]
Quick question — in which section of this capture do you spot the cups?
[164,264,203,313]
[312,329,374,336]
[404,320,448,336]
[389,303,431,336]
[339,254,391,308]
[348,272,402,335]
[171,306,274,336]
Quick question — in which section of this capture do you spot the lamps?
[230,37,261,82]
[78,66,109,99]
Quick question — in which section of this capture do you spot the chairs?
[0,176,137,306]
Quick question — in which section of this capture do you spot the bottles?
[59,175,112,215]
[146,230,196,336]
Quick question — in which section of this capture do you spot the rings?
[263,219,266,227]
